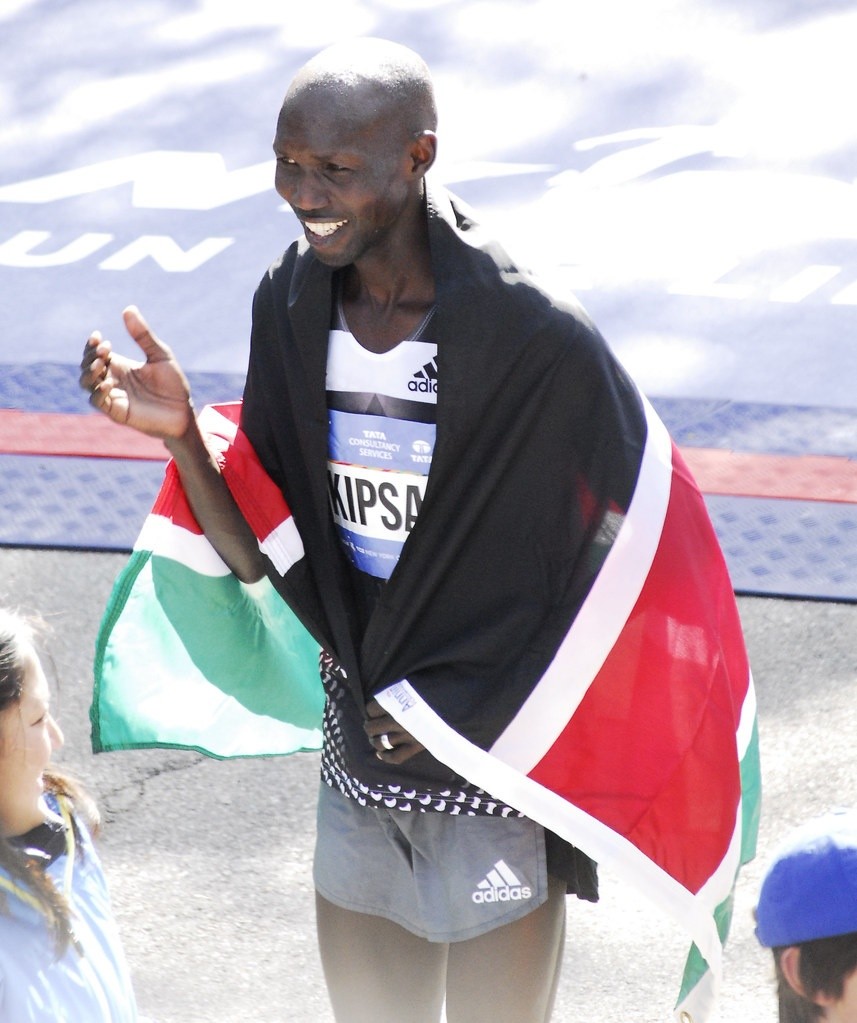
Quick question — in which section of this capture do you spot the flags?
[89,189,760,1023]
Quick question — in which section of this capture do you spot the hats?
[756,831,857,946]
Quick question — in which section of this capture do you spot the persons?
[0,606,136,1023]
[755,829,857,1023]
[76,37,764,1023]
